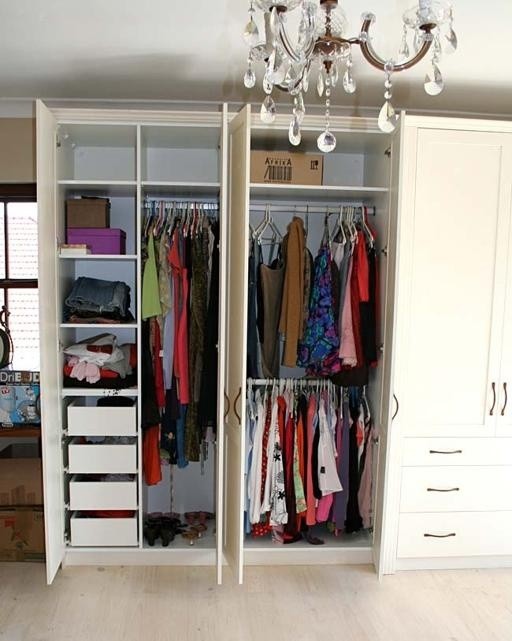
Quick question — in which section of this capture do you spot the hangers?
[140,197,217,241]
[239,370,373,430]
[245,200,377,253]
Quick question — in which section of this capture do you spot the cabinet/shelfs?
[30,96,512,585]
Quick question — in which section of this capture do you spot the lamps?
[240,0,463,153]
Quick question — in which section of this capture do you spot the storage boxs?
[63,195,127,254]
[250,147,326,187]
[0,443,45,563]
